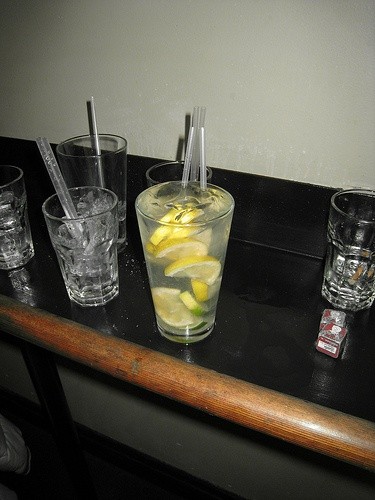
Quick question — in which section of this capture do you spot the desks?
[0,186,374,500]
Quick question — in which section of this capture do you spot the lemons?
[146,207,221,330]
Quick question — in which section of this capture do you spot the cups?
[0,165,35,269]
[322,188,375,312]
[42,186,119,307]
[146,160,212,188]
[135,181,235,343]
[56,134,127,253]
